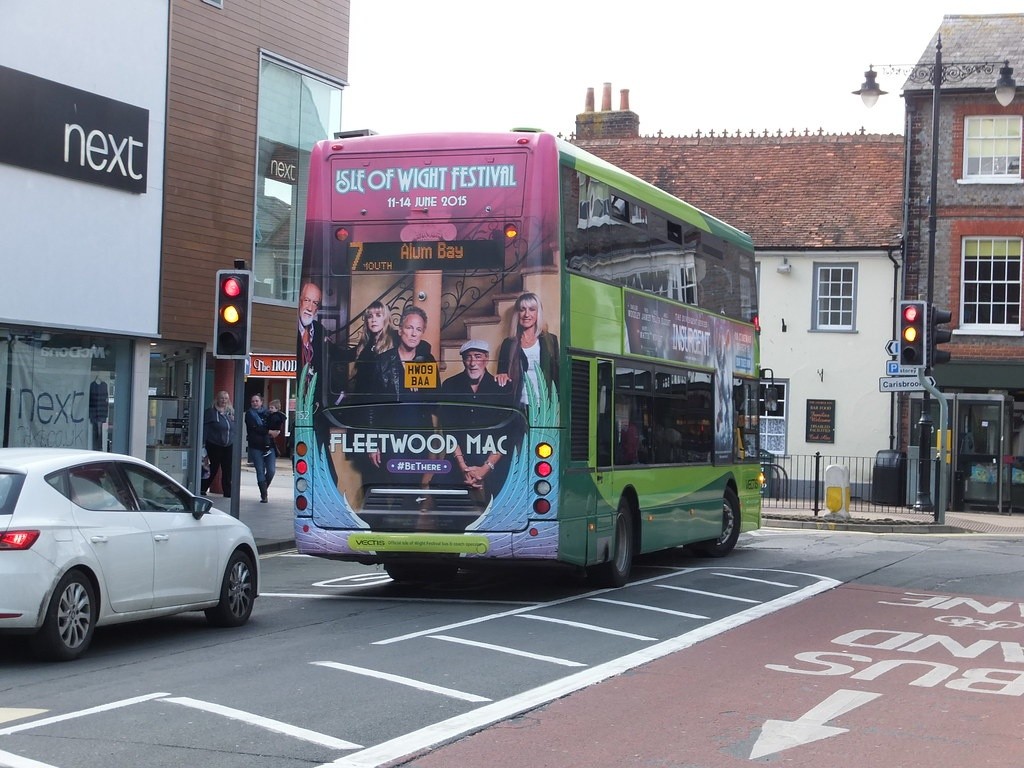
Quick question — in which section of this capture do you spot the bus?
[288,126,779,587]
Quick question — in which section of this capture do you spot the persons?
[201,391,234,497]
[297,281,560,533]
[245,393,287,503]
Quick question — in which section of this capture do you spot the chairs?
[57,477,105,510]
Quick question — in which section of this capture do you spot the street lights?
[849,31,1017,512]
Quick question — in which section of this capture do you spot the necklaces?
[520,334,536,347]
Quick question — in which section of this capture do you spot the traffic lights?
[900,299,927,369]
[211,268,254,359]
[928,306,953,369]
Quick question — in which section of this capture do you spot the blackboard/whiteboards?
[806,399,836,443]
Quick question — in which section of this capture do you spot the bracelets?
[485,459,494,470]
[454,454,466,464]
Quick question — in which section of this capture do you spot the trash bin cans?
[872,450,907,506]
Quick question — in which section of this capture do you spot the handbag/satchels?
[201,448,210,479]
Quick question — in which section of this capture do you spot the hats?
[459,340,490,354]
[666,428,680,444]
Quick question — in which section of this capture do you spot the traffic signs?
[883,339,898,356]
[878,376,937,392]
[885,359,927,376]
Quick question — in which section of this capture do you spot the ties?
[303,326,309,344]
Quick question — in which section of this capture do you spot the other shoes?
[201,491,207,495]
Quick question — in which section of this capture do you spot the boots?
[258,482,268,503]
[266,472,273,488]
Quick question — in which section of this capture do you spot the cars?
[0,446,260,664]
